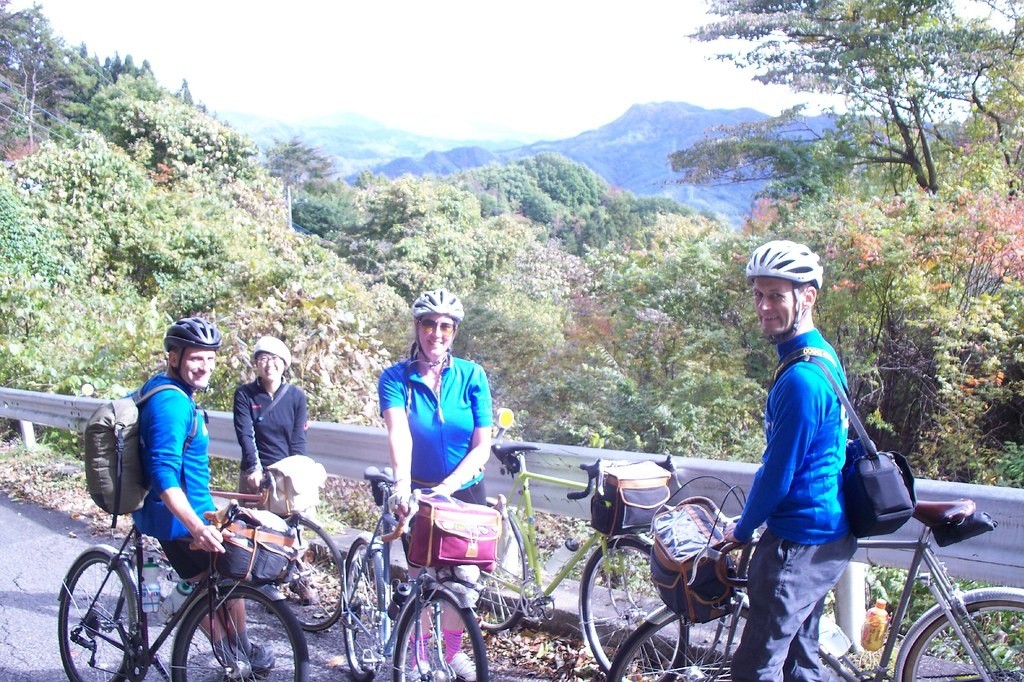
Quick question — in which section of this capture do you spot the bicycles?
[57,467,344,682]
[607,496,1024,682]
[343,465,508,682]
[469,439,689,682]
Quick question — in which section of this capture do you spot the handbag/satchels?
[846,435,876,460]
[842,453,917,538]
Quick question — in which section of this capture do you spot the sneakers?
[289,570,319,604]
[450,652,477,681]
[251,642,275,667]
[224,666,270,682]
[409,661,435,682]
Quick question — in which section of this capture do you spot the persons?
[725,239,857,682]
[126,317,274,678]
[378,288,493,682]
[234,335,321,605]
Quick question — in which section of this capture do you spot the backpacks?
[84,373,197,514]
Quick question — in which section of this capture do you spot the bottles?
[860,598,889,650]
[818,614,852,658]
[387,582,412,621]
[158,580,193,623]
[544,538,579,576]
[141,557,160,614]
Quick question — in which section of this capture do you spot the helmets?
[412,288,465,322]
[747,240,824,289]
[164,317,223,351]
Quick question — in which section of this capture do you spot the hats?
[253,336,292,370]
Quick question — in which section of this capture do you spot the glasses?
[256,355,279,362]
[417,319,457,335]
